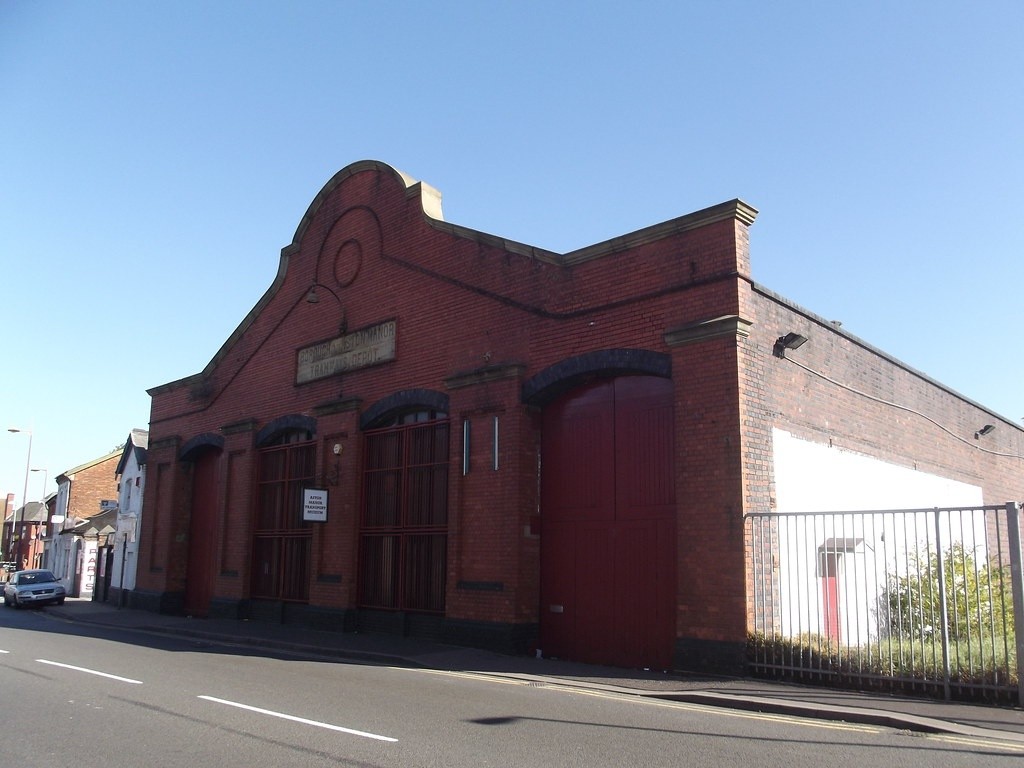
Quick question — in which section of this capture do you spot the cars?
[3,568,67,610]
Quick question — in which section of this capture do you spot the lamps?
[305,284,346,334]
[773,332,808,359]
[975,424,995,440]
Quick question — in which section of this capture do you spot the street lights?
[7,427,34,570]
[30,468,48,502]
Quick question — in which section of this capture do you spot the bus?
[0,561,17,572]
